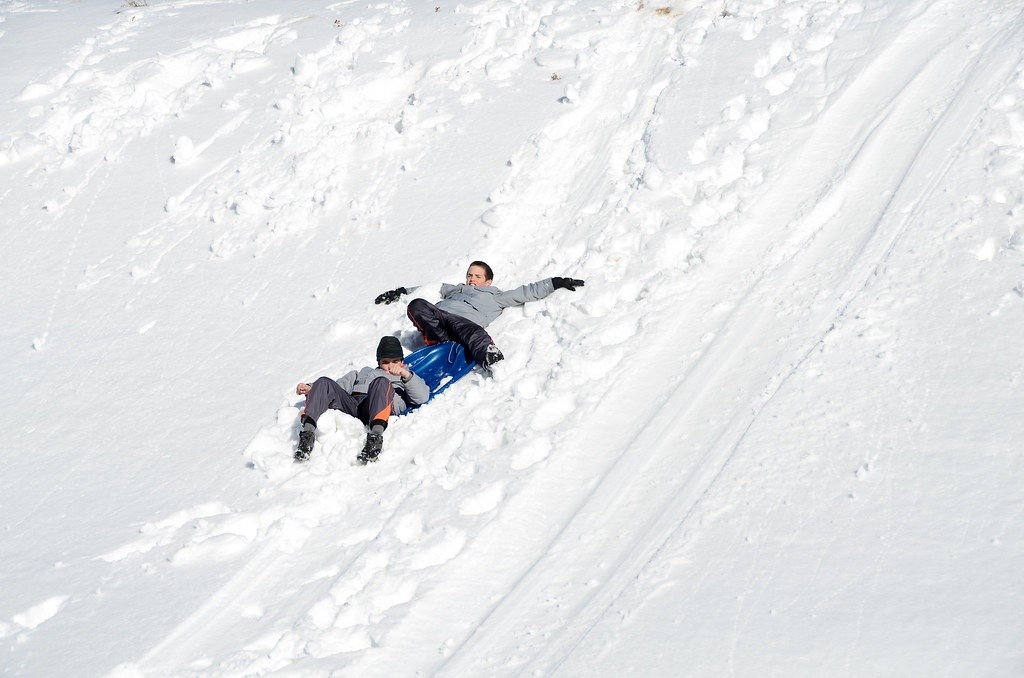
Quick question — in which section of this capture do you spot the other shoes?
[294,431,315,461]
[357,432,383,462]
[483,344,504,372]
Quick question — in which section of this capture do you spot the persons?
[374,261,585,368]
[293,336,430,465]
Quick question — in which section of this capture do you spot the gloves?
[552,277,584,291]
[375,287,407,305]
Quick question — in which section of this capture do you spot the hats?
[377,336,403,361]
